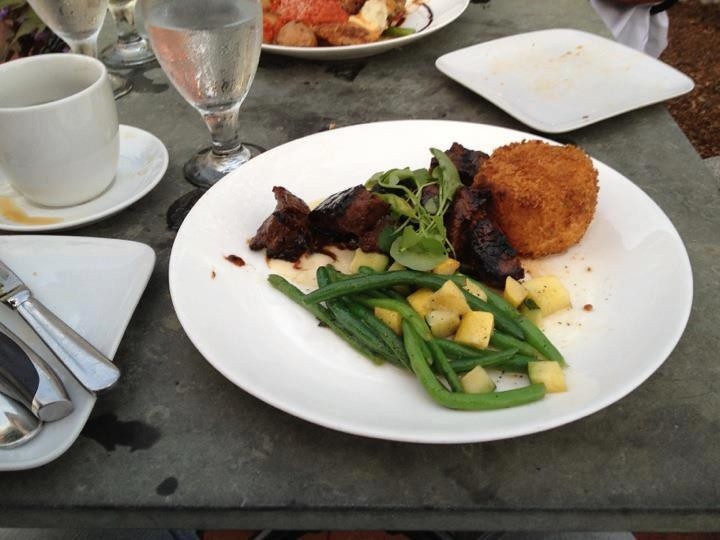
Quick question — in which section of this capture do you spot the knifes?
[73,371,74,374]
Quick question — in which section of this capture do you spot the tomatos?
[260,0,352,45]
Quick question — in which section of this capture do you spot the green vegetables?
[365,145,463,274]
[0,0,71,64]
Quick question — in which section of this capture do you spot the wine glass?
[142,0,268,188]
[97,0,157,68]
[26,0,133,100]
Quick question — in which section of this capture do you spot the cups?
[0,53,119,207]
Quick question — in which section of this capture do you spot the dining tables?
[0,0,720,540]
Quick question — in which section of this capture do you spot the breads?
[476,140,598,258]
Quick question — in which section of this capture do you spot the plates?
[0,235,157,471]
[0,124,169,231]
[261,0,470,61]
[169,119,694,444]
[435,28,695,134]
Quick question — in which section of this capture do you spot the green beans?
[265,266,569,411]
[381,27,415,36]
